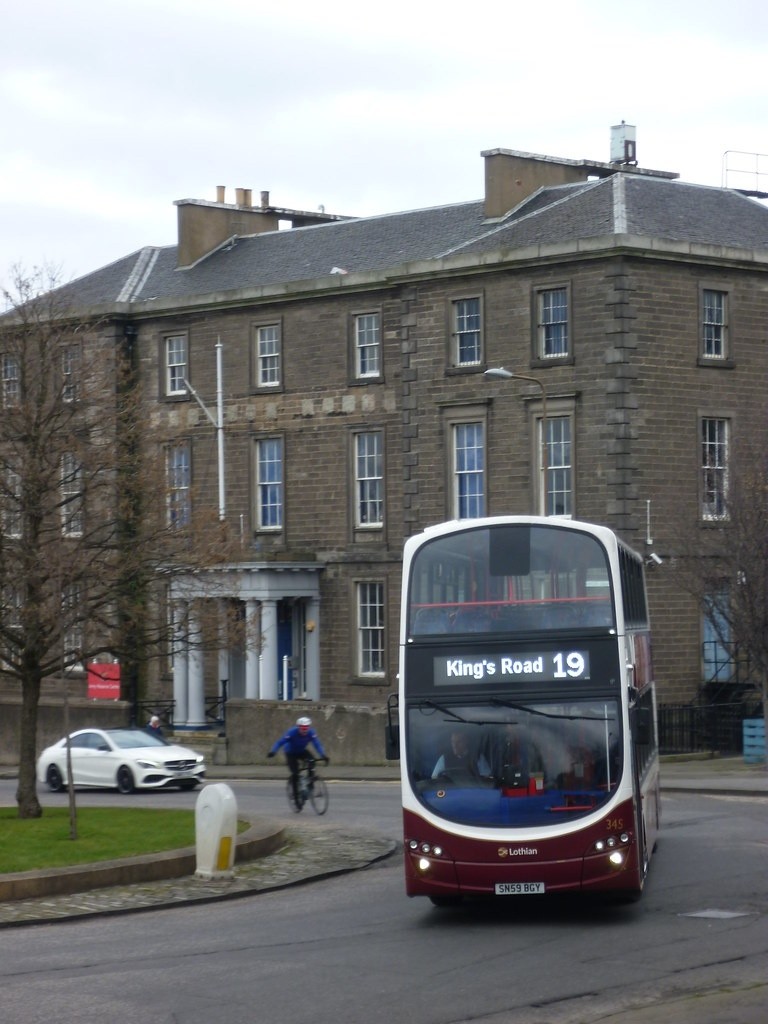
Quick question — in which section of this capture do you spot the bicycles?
[285,754,330,816]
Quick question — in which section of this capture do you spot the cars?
[35,726,209,796]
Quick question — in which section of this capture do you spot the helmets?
[296,717,312,726]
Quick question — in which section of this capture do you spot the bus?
[383,513,661,906]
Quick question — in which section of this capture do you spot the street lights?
[483,366,549,517]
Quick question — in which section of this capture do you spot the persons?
[267,717,329,800]
[431,729,491,780]
[147,716,163,736]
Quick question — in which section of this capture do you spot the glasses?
[300,726,310,730]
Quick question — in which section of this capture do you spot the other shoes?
[295,801,302,810]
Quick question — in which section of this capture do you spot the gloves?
[322,756,329,761]
[268,753,275,757]
